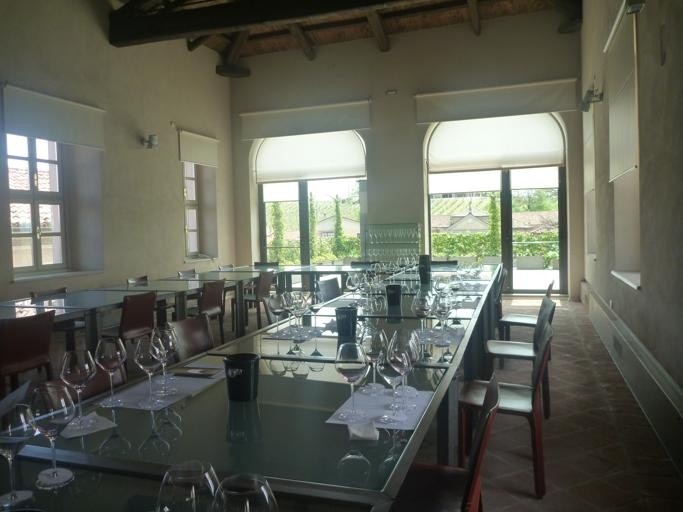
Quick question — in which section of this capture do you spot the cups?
[155,460,224,511]
[226,397,262,442]
[210,474,279,511]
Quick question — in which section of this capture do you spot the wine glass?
[268,251,482,428]
[0,324,180,506]
[332,417,410,483]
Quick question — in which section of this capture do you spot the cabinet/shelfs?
[364,223,421,262]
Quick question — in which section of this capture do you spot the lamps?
[141,135,160,150]
[580,69,603,112]
[625,0,646,15]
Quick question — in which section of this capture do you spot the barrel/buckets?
[226,401,266,444]
[385,285,402,305]
[335,306,357,336]
[419,254,430,272]
[385,305,405,324]
[336,337,359,360]
[419,272,431,283]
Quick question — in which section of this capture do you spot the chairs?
[498,281,554,370]
[487,296,556,418]
[457,320,554,500]
